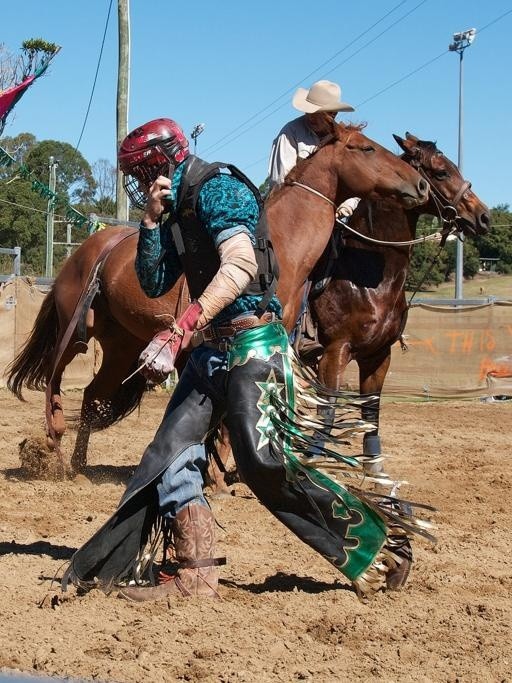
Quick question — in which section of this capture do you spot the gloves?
[138,300,212,387]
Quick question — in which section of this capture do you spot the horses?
[306,129,492,478]
[3,119,431,494]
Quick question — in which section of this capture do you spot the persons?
[267,79,361,360]
[118,117,414,603]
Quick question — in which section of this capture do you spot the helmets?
[116,115,190,183]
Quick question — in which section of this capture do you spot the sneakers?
[298,337,326,360]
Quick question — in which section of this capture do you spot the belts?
[201,310,278,342]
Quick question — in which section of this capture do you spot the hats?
[292,77,356,115]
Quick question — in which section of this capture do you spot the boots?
[118,504,220,604]
[380,515,413,592]
